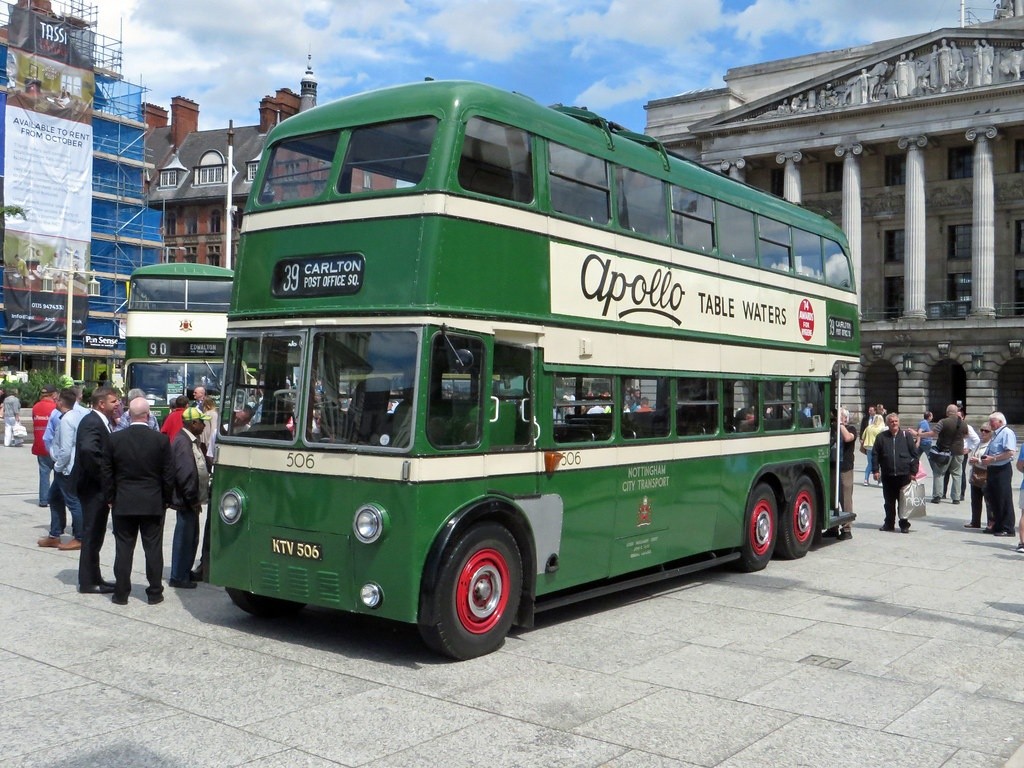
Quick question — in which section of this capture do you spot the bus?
[200,75,864,661]
[124,260,255,431]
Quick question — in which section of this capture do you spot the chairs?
[143,374,165,397]
[347,379,390,449]
[552,400,825,443]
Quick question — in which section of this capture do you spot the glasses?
[980,429,991,433]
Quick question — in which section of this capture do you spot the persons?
[0,369,403,550]
[192,402,254,581]
[102,397,176,604]
[56,86,71,109]
[72,387,120,594]
[745,37,995,121]
[15,250,60,289]
[555,390,1024,553]
[171,408,209,592]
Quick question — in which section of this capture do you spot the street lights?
[35,245,102,383]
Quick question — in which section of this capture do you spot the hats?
[9,388,19,395]
[54,374,75,390]
[181,407,213,421]
[40,384,58,393]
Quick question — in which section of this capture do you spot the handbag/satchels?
[12,422,28,437]
[929,445,951,464]
[899,478,927,519]
[858,439,866,454]
[969,467,988,488]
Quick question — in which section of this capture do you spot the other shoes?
[964,523,1024,553]
[111,593,128,605]
[58,539,83,551]
[837,528,852,540]
[169,570,203,588]
[863,480,870,486]
[953,500,961,504]
[900,527,909,533]
[931,497,940,503]
[821,526,839,537]
[37,537,60,547]
[79,580,116,593]
[879,526,895,530]
[148,594,164,604]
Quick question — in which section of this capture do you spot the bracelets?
[917,432,919,437]
[994,456,996,462]
